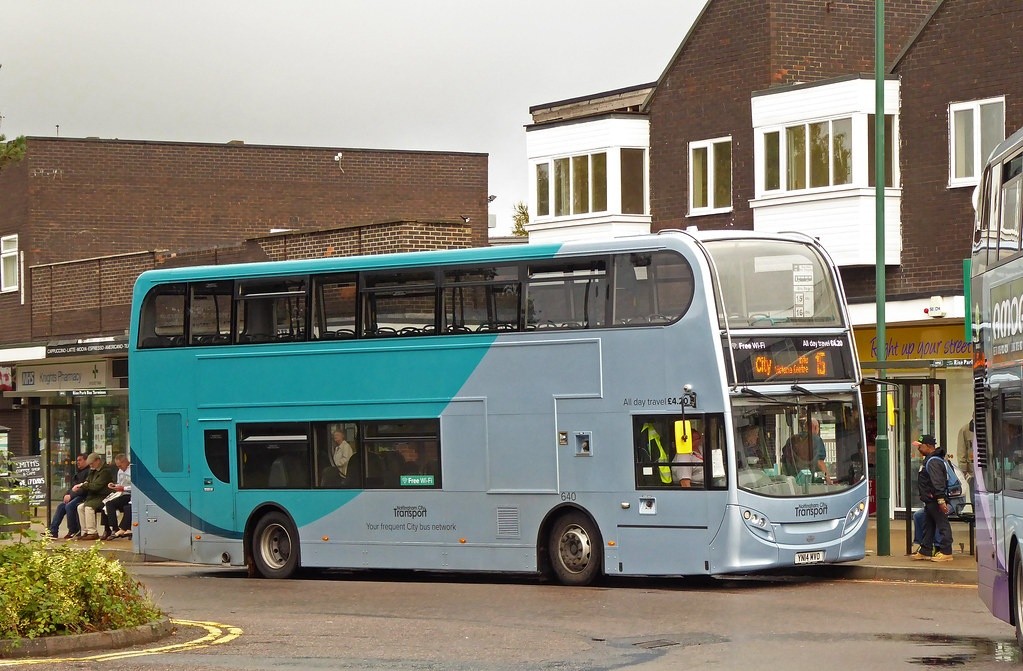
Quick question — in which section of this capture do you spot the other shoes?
[79,529,131,540]
[41,533,57,540]
[64,531,80,540]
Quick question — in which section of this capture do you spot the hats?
[913,436,936,445]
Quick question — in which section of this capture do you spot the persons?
[71,452,114,541]
[955,413,976,527]
[111,503,132,541]
[741,423,772,469]
[331,432,353,479]
[40,453,92,540]
[670,426,727,488]
[101,453,131,541]
[782,418,831,485]
[912,434,954,561]
[911,465,967,554]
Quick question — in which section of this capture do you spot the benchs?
[943,512,975,556]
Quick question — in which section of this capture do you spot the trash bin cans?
[0,487,30,533]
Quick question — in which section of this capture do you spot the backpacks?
[926,457,962,498]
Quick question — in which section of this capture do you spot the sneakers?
[910,553,931,561]
[931,551,953,562]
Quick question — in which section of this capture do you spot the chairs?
[142,312,787,347]
[220,451,424,491]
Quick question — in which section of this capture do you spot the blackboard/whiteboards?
[9,456,47,507]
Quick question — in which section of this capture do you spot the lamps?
[461,215,470,223]
[488,194,497,202]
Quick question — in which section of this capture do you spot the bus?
[129,226,898,590]
[962,122,1023,657]
